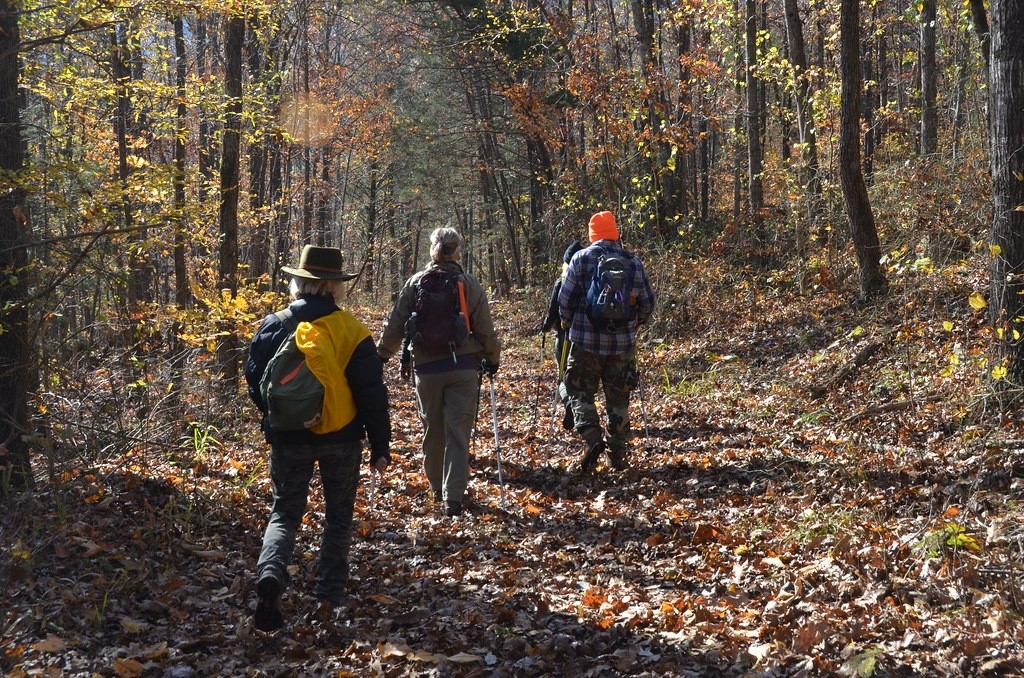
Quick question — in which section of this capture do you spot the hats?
[588,211,618,240]
[282,244,359,282]
[563,241,585,264]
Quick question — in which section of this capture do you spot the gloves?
[561,320,570,330]
[380,357,389,364]
[482,358,499,374]
[399,361,411,382]
[541,324,552,332]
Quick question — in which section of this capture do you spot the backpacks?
[259,304,335,431]
[412,269,470,355]
[579,245,634,334]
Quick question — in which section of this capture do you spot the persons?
[541,212,654,470]
[246,244,391,630]
[377,226,501,515]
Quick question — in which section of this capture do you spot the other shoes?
[315,589,339,611]
[254,564,284,631]
[445,500,462,516]
[431,489,443,504]
[582,433,606,469]
[563,403,574,430]
[607,449,626,471]
